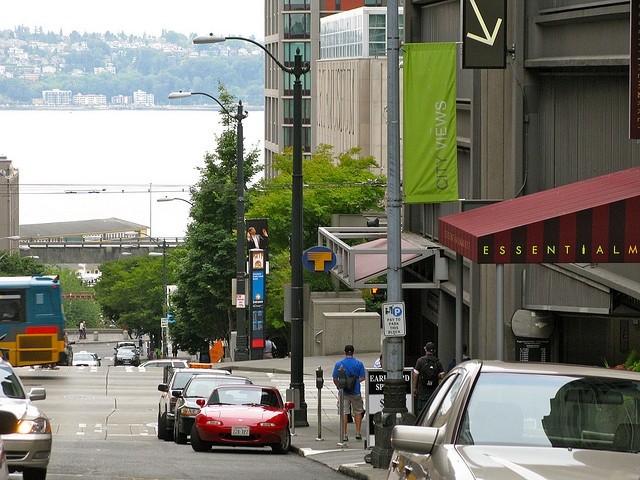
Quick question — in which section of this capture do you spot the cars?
[72,350,101,366]
[0,356,51,479]
[387,358,640,480]
[190,384,295,452]
[158,367,230,440]
[172,375,253,443]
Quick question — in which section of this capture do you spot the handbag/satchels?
[271,342,278,357]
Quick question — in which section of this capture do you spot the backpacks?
[420,357,439,391]
[341,360,359,393]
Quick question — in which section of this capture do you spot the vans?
[137,358,188,367]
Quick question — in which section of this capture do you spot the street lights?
[168,90,248,361]
[156,195,204,223]
[193,32,308,426]
[125,230,167,356]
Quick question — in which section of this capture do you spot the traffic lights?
[370,282,386,296]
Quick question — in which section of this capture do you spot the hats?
[425,342,435,351]
[344,345,354,352]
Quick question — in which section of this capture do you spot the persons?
[412,341,445,416]
[139,337,144,356]
[246,227,264,256]
[260,225,268,248]
[333,345,366,440]
[374,355,383,368]
[172,340,178,358]
[136,347,141,360]
[446,344,471,374]
[262,335,277,359]
[79,321,88,340]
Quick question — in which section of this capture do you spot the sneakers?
[355,432,362,439]
[343,434,348,440]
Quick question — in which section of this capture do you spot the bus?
[0,274,65,366]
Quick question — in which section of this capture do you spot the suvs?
[114,346,139,365]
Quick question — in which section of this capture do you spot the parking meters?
[316,365,323,437]
[337,364,346,442]
